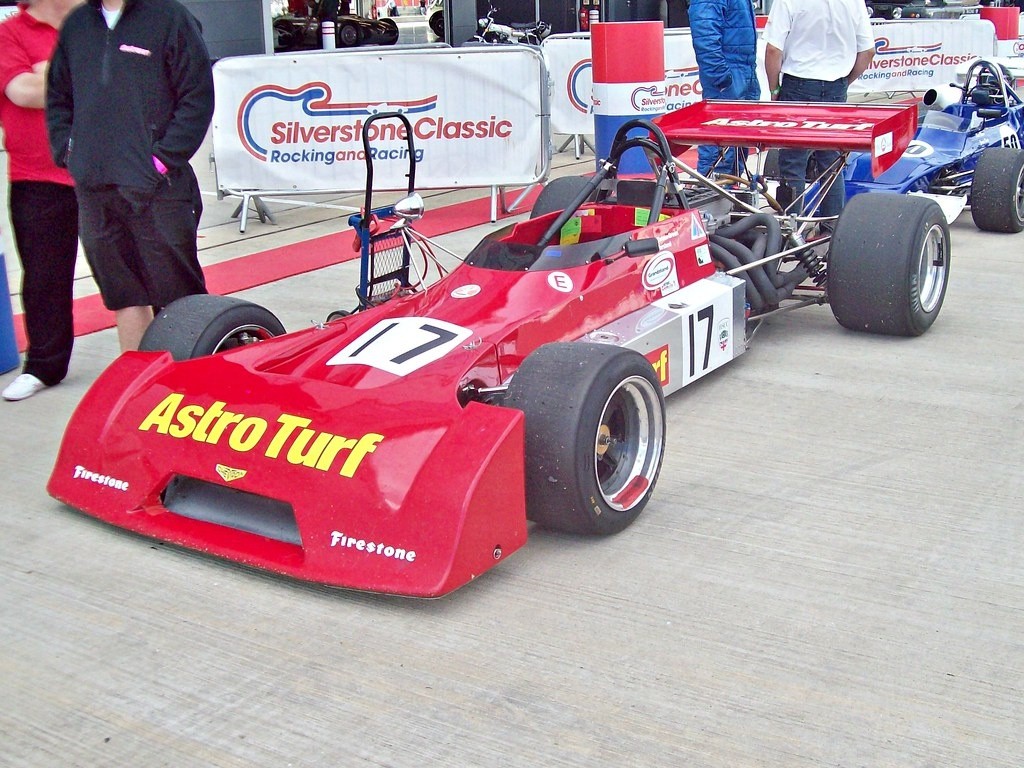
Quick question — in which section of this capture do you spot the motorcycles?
[468,0,551,46]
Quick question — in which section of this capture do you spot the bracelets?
[771,85,780,94]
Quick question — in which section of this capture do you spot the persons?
[688,0,761,189]
[287,0,351,49]
[45,0,216,354]
[0,0,84,402]
[761,0,876,233]
[419,0,426,15]
[386,0,399,17]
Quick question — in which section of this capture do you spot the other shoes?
[804,221,838,242]
[2,372,50,400]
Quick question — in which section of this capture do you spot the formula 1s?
[45,98,952,601]
[271,5,398,47]
[804,59,1024,234]
